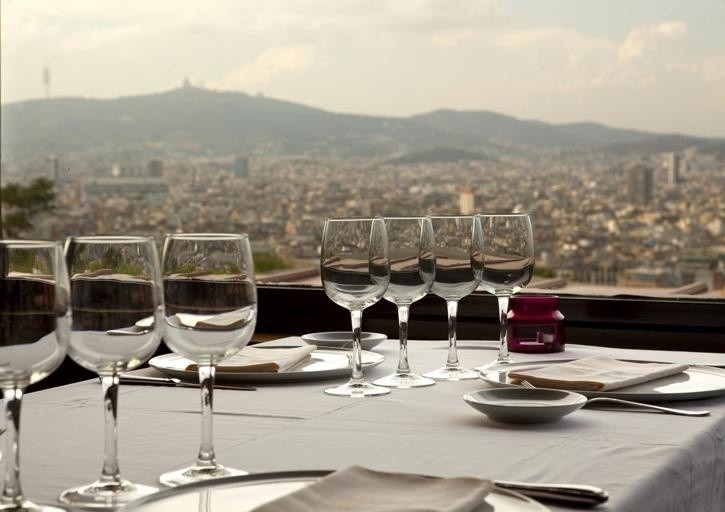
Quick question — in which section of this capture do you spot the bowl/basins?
[148,347,384,385]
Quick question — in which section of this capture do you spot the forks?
[519,381,711,419]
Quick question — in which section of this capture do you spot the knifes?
[98,373,257,394]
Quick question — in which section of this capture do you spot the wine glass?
[61,234,166,507]
[319,215,533,398]
[1,237,69,512]
[159,232,259,487]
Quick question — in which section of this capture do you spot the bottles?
[507,294,565,356]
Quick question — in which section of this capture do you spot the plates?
[301,330,387,352]
[465,386,586,425]
[116,471,552,512]
[478,356,724,400]
